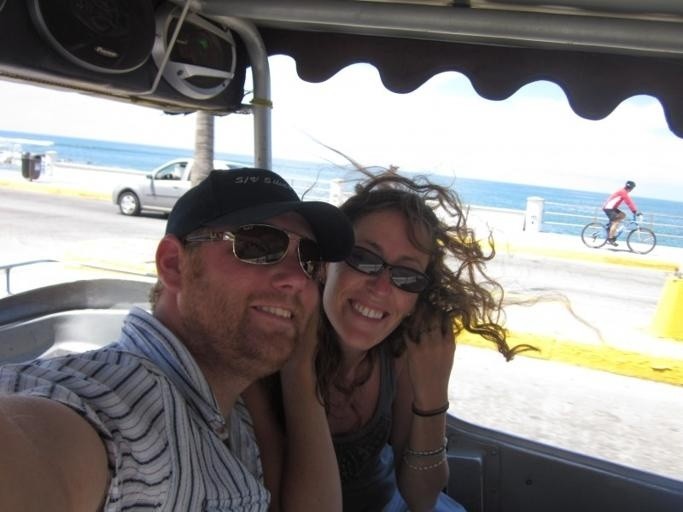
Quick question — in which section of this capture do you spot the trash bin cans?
[22,152,41,181]
[526,195,544,236]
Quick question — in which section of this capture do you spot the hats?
[166,167,355,263]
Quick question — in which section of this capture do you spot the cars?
[113,158,255,220]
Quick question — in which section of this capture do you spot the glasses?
[187,221,323,283]
[344,245,431,294]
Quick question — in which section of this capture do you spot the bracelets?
[397,435,449,473]
[408,400,449,417]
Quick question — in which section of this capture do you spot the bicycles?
[581,211,656,254]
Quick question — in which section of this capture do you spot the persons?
[239,167,540,510]
[0,164,355,511]
[602,180,638,248]
